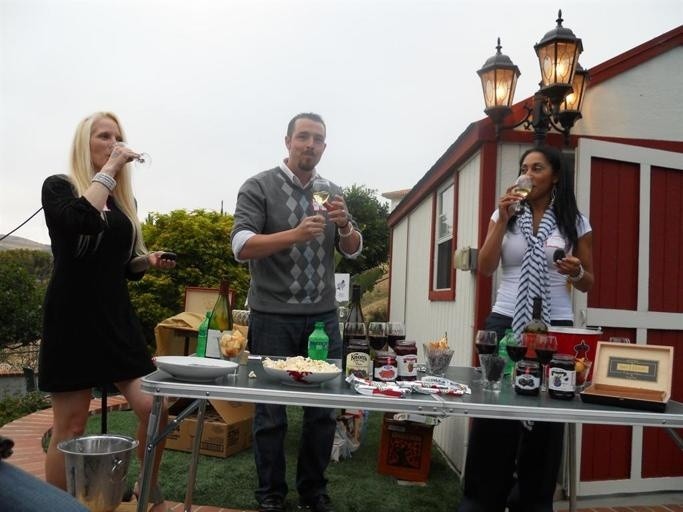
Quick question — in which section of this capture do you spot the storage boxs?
[159,388,255,459]
[375,411,436,483]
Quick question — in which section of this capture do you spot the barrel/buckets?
[55,431,140,510]
[518,326,605,385]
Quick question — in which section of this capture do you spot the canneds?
[345,338,370,384]
[373,350,398,382]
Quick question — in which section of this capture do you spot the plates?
[260,364,342,390]
[152,355,238,383]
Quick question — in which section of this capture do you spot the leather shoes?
[259,497,287,512]
[298,494,337,512]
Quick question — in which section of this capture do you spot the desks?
[137,349,683,512]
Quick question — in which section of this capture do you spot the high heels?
[133,475,173,512]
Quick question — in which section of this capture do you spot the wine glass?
[311,179,330,234]
[388,323,405,351]
[507,334,530,387]
[369,322,386,352]
[113,140,152,170]
[476,331,499,387]
[533,334,557,391]
[511,173,534,215]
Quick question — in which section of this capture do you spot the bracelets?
[569,265,585,283]
[338,212,353,230]
[337,222,354,238]
[89,172,117,191]
[144,250,154,266]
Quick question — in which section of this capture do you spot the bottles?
[373,355,398,383]
[205,273,234,358]
[196,312,217,356]
[499,330,513,374]
[309,322,329,363]
[342,284,370,380]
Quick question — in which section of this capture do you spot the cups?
[423,344,454,377]
[479,352,504,394]
[576,361,594,389]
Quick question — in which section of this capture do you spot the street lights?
[476,6,585,148]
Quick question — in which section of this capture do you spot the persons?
[460,145,594,511]
[232,113,363,509]
[38,109,175,512]
